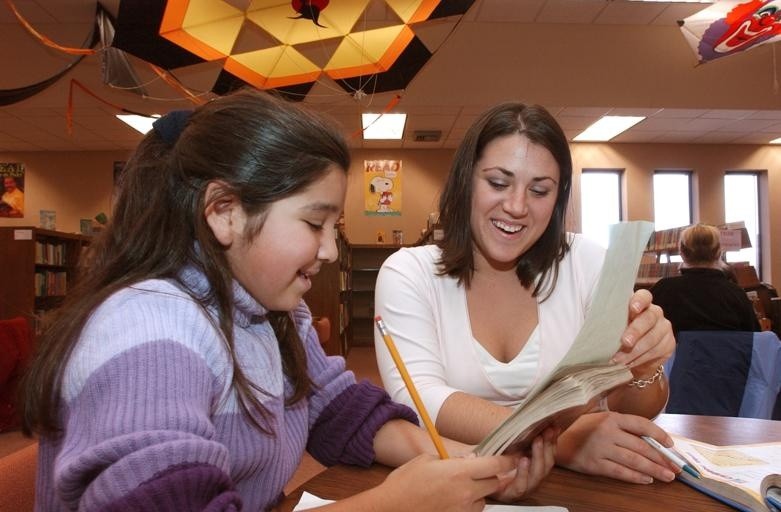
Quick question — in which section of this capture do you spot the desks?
[258,410,781,512]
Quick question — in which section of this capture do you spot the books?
[339,234,351,357]
[474,361,633,458]
[669,434,781,510]
[638,227,761,290]
[35,237,94,334]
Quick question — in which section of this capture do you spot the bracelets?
[627,367,665,388]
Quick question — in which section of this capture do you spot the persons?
[655,225,762,342]
[26,89,563,512]
[374,102,684,486]
[2,175,24,214]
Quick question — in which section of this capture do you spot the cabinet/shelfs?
[631,220,780,328]
[346,237,418,350]
[305,225,354,360]
[0,224,93,345]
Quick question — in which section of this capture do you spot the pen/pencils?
[642,436,703,481]
[374,314,449,459]
